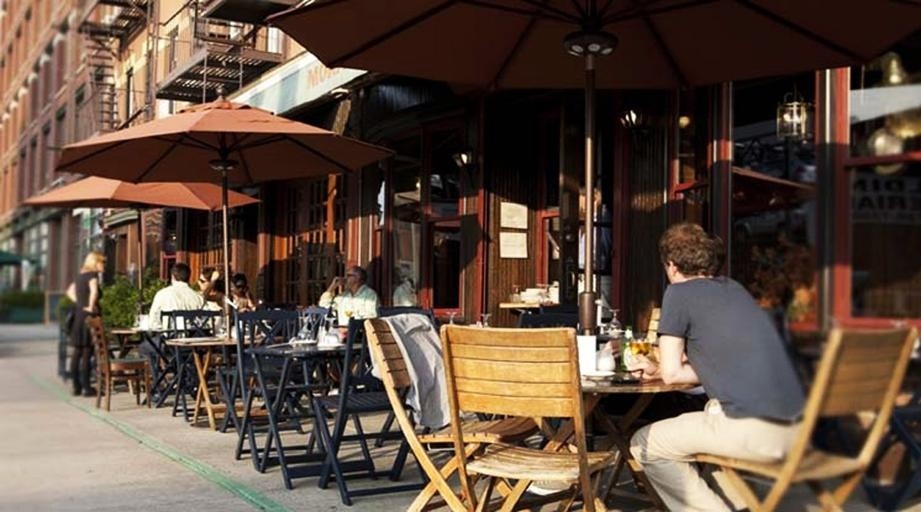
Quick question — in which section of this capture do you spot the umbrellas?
[20,173,265,287]
[263,1,921,340]
[55,96,399,325]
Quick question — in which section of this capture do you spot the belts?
[763,416,804,427]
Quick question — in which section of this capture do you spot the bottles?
[510,279,563,305]
[290,316,345,350]
[574,309,655,378]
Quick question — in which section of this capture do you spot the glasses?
[233,283,246,288]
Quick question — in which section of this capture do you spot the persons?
[315,264,379,335]
[227,274,256,317]
[628,222,808,511]
[65,251,109,396]
[135,261,206,363]
[193,265,229,329]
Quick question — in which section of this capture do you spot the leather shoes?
[75,387,103,396]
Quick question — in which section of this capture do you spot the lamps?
[451,148,478,196]
[855,50,919,175]
[777,83,813,145]
[616,106,658,142]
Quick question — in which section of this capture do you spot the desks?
[240,338,377,491]
[161,331,283,432]
[575,367,702,512]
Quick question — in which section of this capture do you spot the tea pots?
[134,313,150,330]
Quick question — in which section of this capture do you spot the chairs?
[691,323,920,510]
[87,313,151,412]
[146,303,439,512]
[362,313,545,511]
[439,324,617,512]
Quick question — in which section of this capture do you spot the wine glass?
[481,313,493,327]
[445,310,459,325]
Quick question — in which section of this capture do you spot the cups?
[213,322,242,341]
